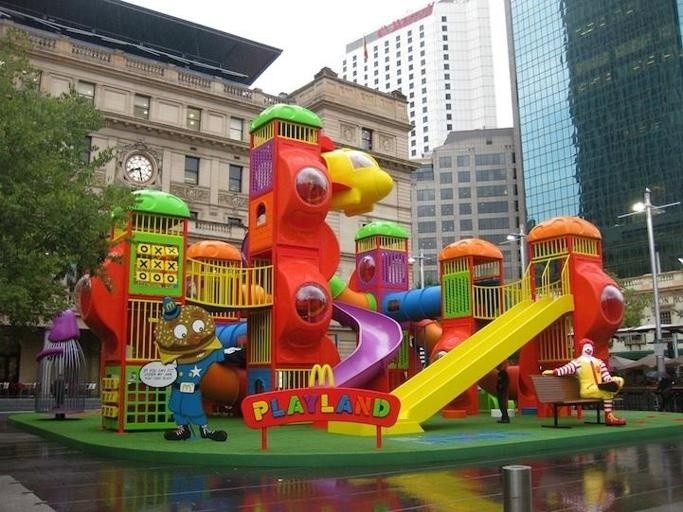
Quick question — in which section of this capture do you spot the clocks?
[122,150,159,187]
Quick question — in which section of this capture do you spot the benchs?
[529,374,624,429]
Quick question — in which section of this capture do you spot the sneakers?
[497,420,509,423]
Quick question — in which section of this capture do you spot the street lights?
[407,247,431,290]
[505,223,525,278]
[632,186,663,385]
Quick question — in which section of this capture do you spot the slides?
[313,301,403,388]
[327,293,575,437]
